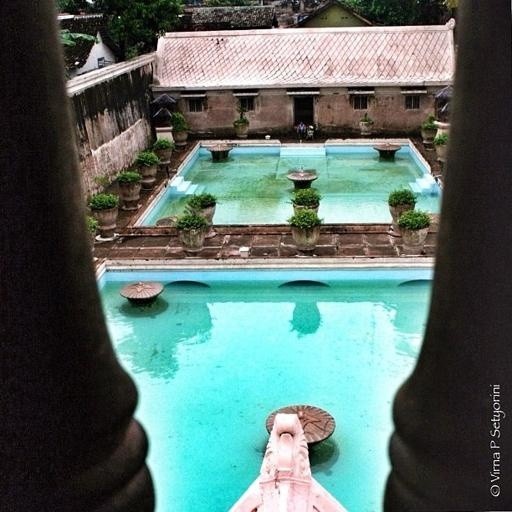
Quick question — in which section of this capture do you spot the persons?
[296,120,307,142]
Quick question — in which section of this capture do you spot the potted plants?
[87,139,174,257]
[289,188,322,214]
[169,112,190,146]
[184,193,217,239]
[359,113,374,136]
[233,105,249,137]
[421,112,438,151]
[433,132,448,163]
[397,210,430,256]
[286,209,324,251]
[388,189,418,237]
[176,215,208,253]
